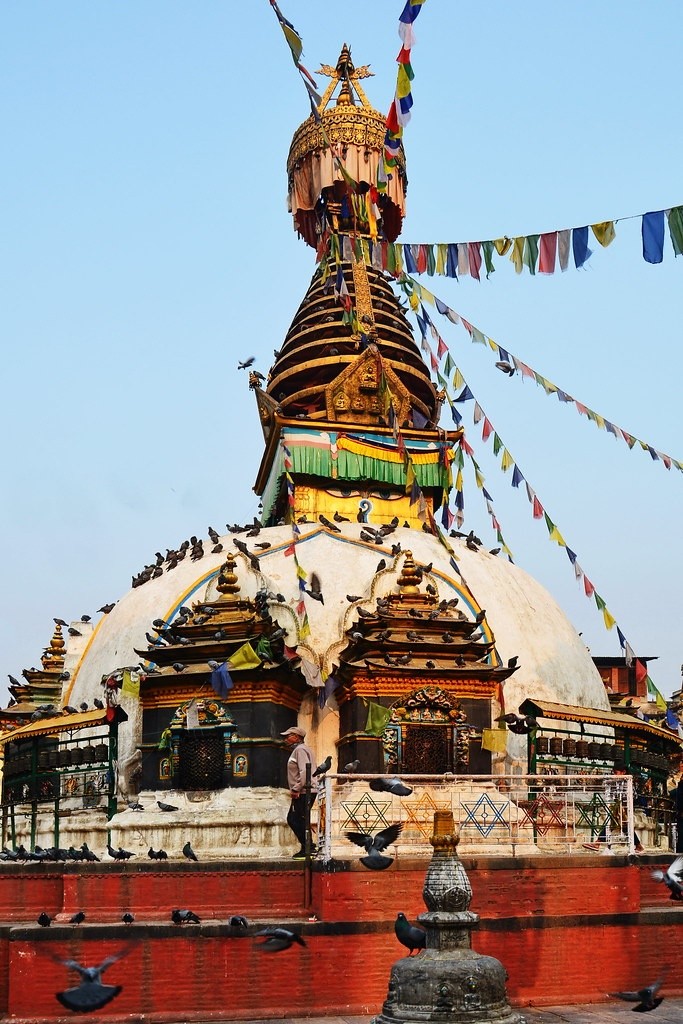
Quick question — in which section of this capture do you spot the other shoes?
[582,843,600,851]
[292,848,318,860]
[634,845,644,853]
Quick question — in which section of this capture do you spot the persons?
[280,727,319,860]
[582,763,645,853]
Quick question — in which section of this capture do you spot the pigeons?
[33,939,138,1013]
[343,822,404,871]
[67,910,86,926]
[250,926,310,949]
[122,912,134,926]
[230,914,248,929]
[37,911,52,927]
[311,756,333,778]
[608,964,669,1013]
[237,275,415,427]
[651,854,683,901]
[0,841,200,863]
[126,799,144,812]
[394,912,427,958]
[2,506,543,735]
[171,909,200,927]
[369,776,413,797]
[340,759,361,774]
[157,801,178,811]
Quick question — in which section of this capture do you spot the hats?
[280,727,306,737]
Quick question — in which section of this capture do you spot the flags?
[271,1,683,738]
